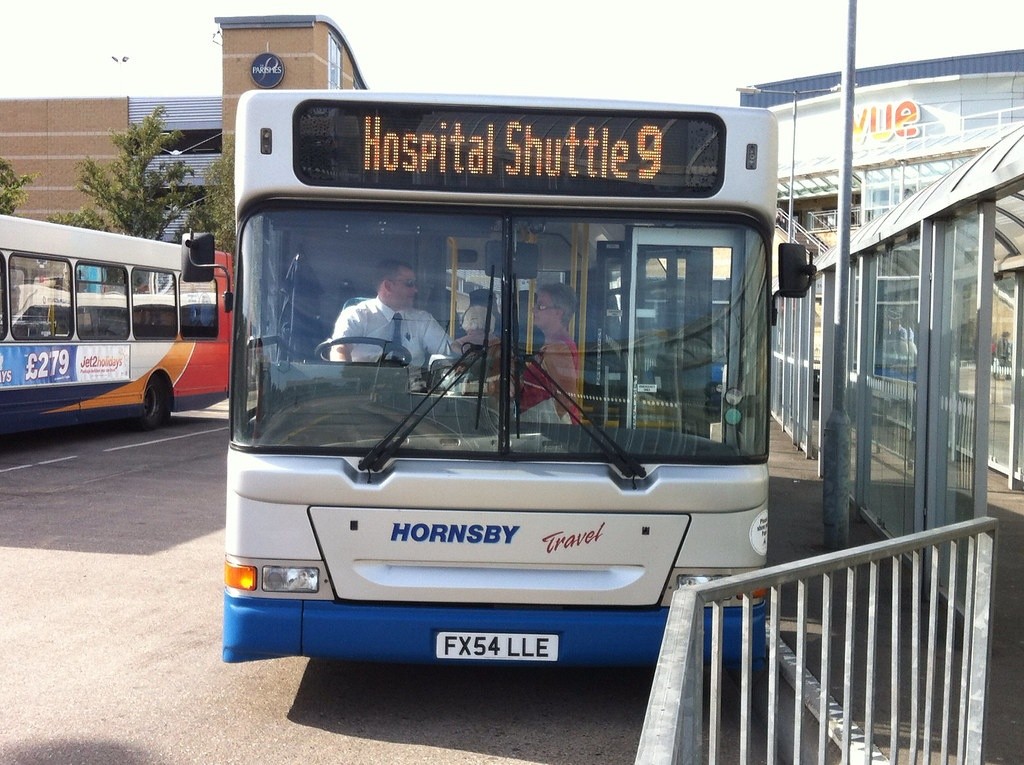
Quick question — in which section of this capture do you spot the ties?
[392,313,403,362]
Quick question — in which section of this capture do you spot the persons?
[330,259,466,392]
[518,282,582,424]
[451,305,516,397]
[996,332,1012,366]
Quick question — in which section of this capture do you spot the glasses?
[386,280,416,288]
[535,303,561,311]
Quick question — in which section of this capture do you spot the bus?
[177,90,817,667]
[0,209,235,440]
